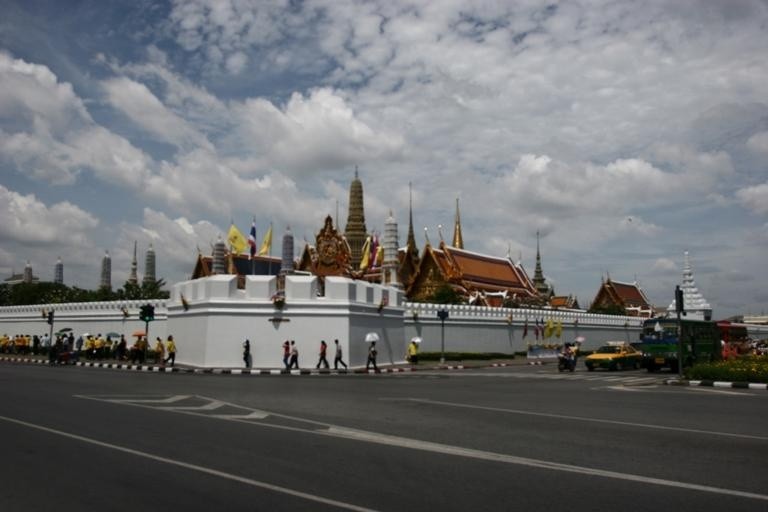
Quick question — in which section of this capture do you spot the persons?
[242,339,250,367]
[290,340,299,368]
[317,340,329,368]
[564,342,581,360]
[0,332,177,366]
[334,339,347,369]
[366,341,378,369]
[282,340,290,368]
[408,342,419,368]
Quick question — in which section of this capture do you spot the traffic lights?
[140,306,155,321]
[47,312,53,324]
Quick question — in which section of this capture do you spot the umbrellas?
[364,332,380,342]
[411,336,424,343]
[60,327,73,331]
[574,336,586,342]
[132,330,146,336]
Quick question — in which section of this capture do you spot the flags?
[227,224,248,258]
[248,219,257,258]
[359,236,384,269]
[258,226,272,256]
[521,317,562,340]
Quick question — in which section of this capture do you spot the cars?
[585,341,643,371]
[752,339,768,356]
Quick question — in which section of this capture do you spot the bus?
[717,319,752,359]
[640,319,723,372]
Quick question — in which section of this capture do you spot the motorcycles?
[556,352,576,372]
